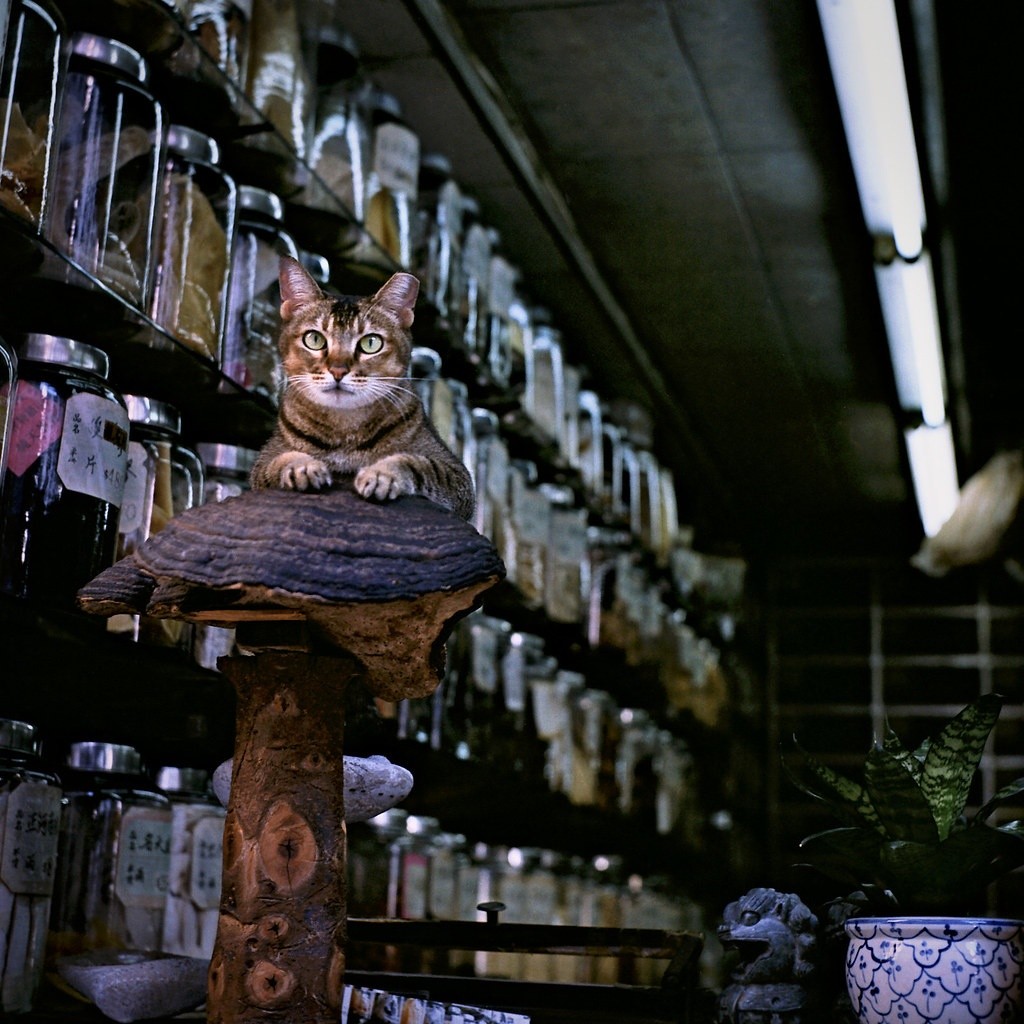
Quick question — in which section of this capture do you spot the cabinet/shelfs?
[1,0,745,1024]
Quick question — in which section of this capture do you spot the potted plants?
[792,688,1023,1024]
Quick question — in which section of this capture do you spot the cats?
[249,252,478,522]
